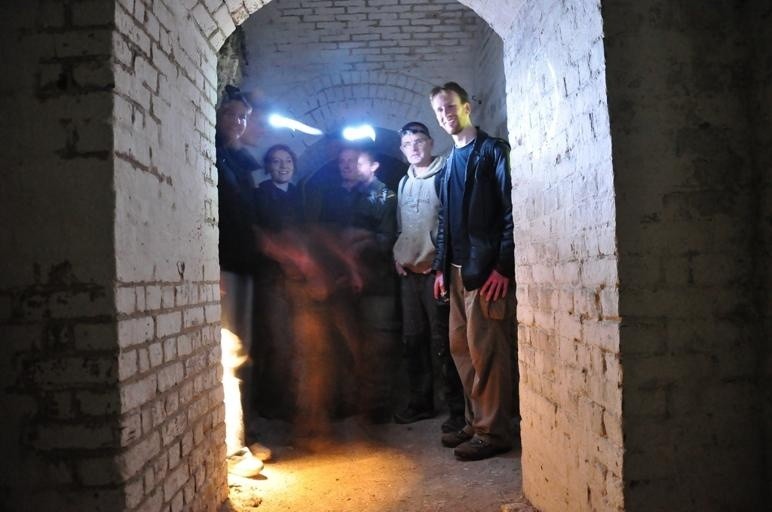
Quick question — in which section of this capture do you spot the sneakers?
[442,414,512,461]
[225,440,271,477]
[394,404,435,424]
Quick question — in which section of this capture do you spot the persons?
[249,142,365,425]
[427,81,518,460]
[389,121,468,432]
[322,143,401,420]
[218,83,294,478]
